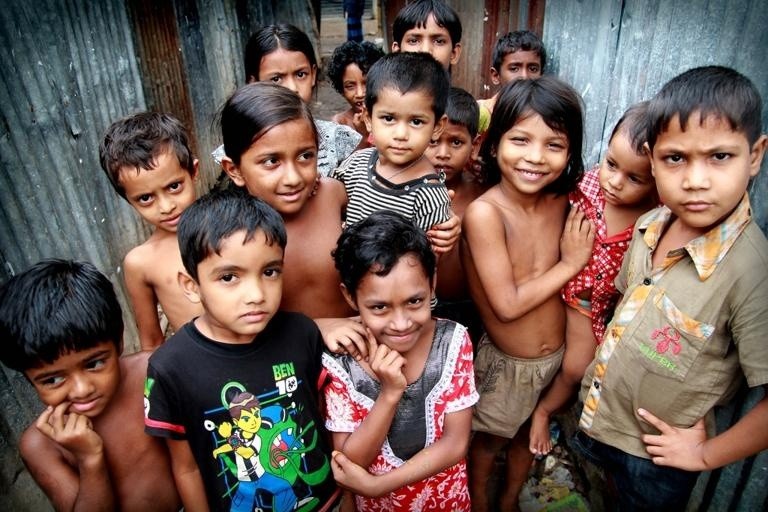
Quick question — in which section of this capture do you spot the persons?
[143,0,768,511]
[0,256,181,512]
[98,111,207,352]
